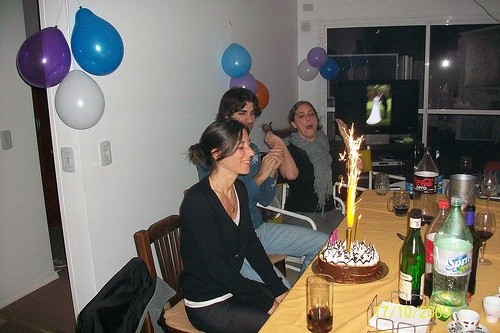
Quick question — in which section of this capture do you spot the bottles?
[434,150,442,195]
[398,196,481,322]
[412,146,439,226]
[405,143,420,198]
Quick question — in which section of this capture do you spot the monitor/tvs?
[336,79,420,144]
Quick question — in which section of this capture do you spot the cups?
[375,173,389,195]
[480,167,498,197]
[452,309,480,333]
[482,294,500,323]
[387,191,410,216]
[306,274,334,333]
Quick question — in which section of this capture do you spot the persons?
[262,101,345,236]
[197,86,331,292]
[366,90,388,124]
[180,118,289,333]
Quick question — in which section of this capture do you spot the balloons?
[16,27,71,88]
[254,80,269,110]
[71,9,124,76]
[320,58,339,80]
[55,70,105,130]
[230,72,257,94]
[297,58,318,81]
[222,43,251,78]
[307,48,326,67]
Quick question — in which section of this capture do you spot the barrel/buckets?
[444,174,477,210]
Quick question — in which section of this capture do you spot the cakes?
[318,238,380,281]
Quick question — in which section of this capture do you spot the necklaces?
[210,176,235,213]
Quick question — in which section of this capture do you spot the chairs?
[256,181,367,232]
[79,257,169,333]
[134,212,208,333]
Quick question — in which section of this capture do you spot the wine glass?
[474,210,497,266]
[460,155,472,174]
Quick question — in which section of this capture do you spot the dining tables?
[257,189,500,333]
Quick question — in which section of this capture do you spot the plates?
[447,321,489,333]
